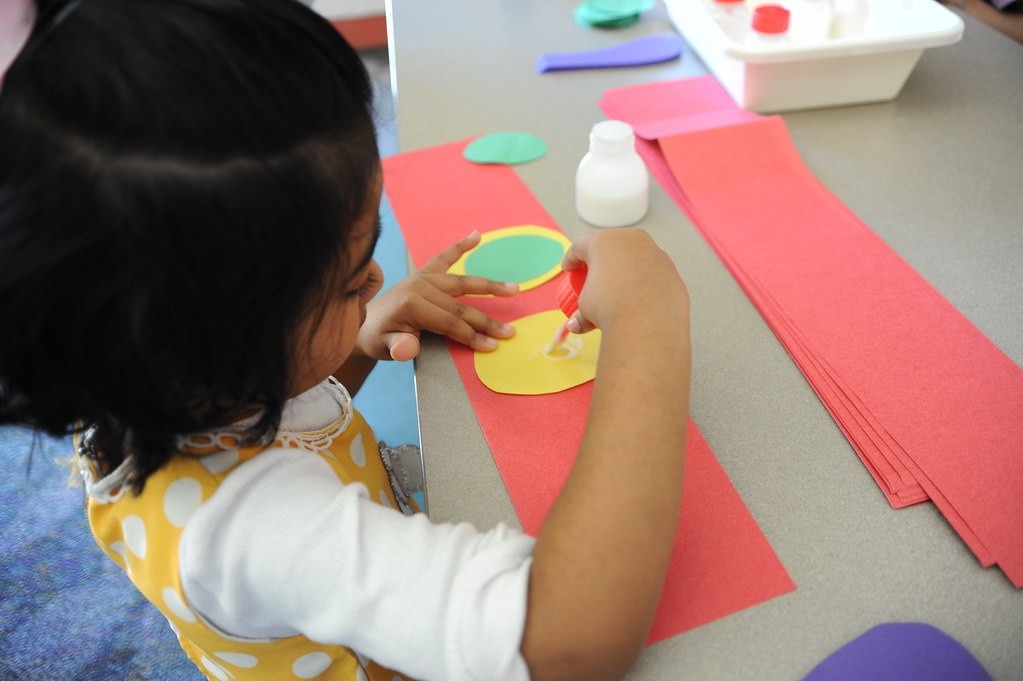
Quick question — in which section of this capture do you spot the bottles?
[576,120,649,226]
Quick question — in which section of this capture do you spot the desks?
[386,0,1023,681]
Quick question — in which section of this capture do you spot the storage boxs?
[665,0,965,114]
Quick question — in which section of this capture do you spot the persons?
[0,0,693,681]
